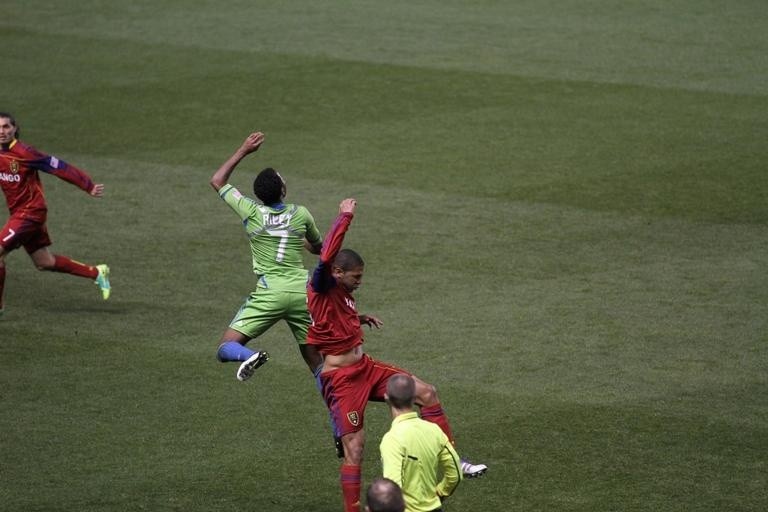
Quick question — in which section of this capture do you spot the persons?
[365,478,405,512]
[1,110,115,307]
[210,129,348,457]
[305,198,489,512]
[380,373,463,511]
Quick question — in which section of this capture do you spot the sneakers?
[235,350,271,383]
[93,261,112,301]
[461,459,489,479]
